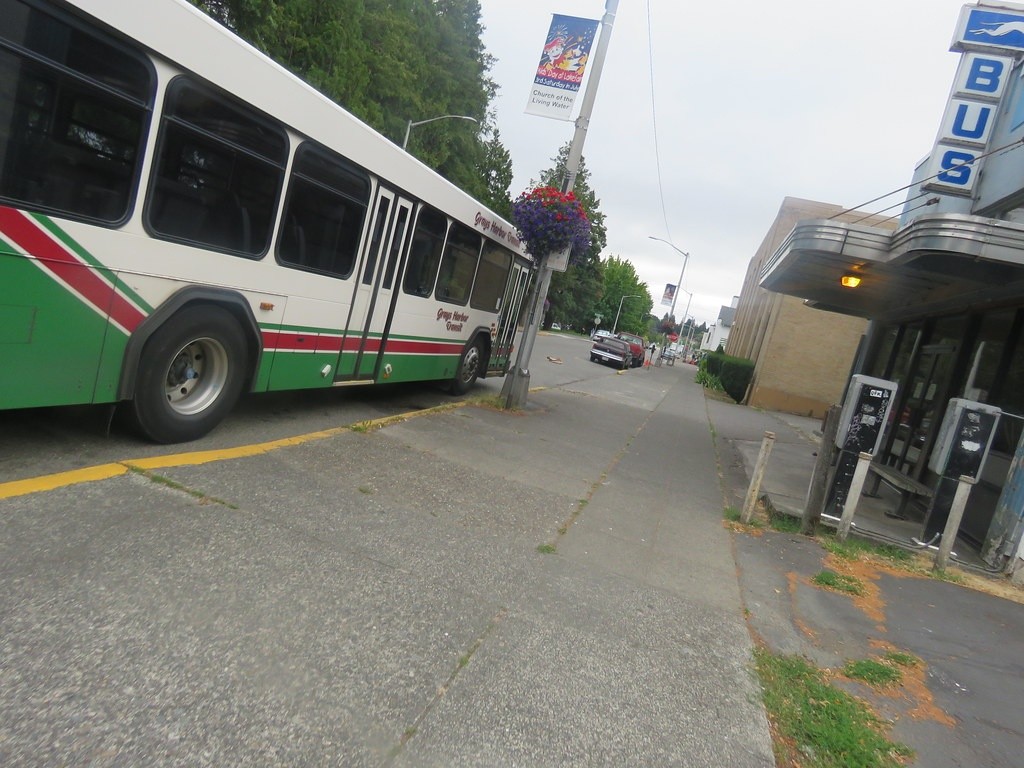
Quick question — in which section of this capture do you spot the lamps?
[842,272,863,288]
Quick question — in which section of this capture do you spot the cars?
[551,323,561,331]
[592,330,610,341]
[590,336,632,369]
[617,332,646,366]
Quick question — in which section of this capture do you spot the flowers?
[512,185,592,268]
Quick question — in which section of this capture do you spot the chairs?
[214,190,251,252]
[266,207,306,265]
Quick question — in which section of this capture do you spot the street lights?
[611,294,642,338]
[649,235,689,367]
[403,114,477,153]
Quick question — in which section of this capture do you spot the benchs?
[862,464,933,520]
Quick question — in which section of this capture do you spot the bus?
[0,2,540,446]
